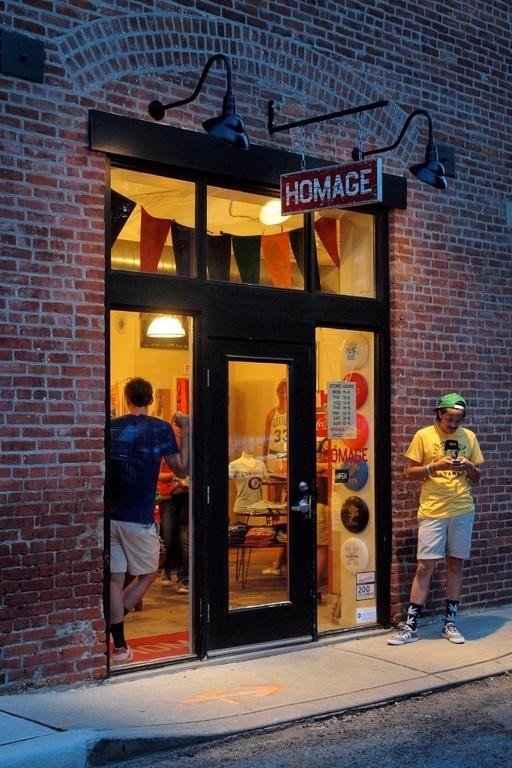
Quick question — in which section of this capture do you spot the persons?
[157,416,189,595]
[386,393,487,645]
[109,377,190,667]
[317,502,329,601]
[263,380,288,526]
[261,542,287,576]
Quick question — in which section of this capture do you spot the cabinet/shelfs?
[230,477,289,591]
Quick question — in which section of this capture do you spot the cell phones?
[453,461,460,466]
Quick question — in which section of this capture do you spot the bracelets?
[424,464,432,476]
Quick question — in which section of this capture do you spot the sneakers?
[175,580,190,596]
[386,623,422,644]
[160,569,172,586]
[113,644,134,667]
[261,566,282,576]
[441,622,467,645]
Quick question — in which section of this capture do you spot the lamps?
[145,317,184,341]
[153,50,250,152]
[260,200,287,229]
[357,108,446,193]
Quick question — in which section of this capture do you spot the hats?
[432,393,469,413]
